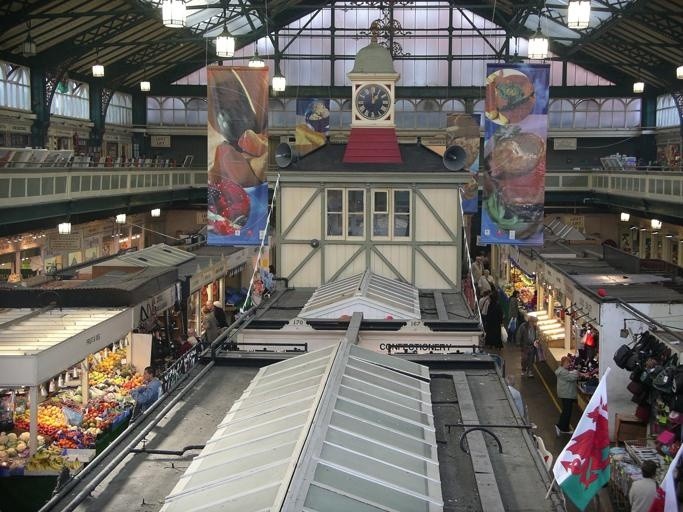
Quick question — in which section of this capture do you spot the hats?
[213,301,222,309]
[528,316,539,322]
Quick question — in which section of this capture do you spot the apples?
[87,370,146,389]
[14,417,59,434]
[89,400,119,418]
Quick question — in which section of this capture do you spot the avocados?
[81,417,97,429]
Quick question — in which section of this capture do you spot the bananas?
[24,443,80,472]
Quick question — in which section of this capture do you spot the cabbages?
[65,386,92,403]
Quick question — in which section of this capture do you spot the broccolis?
[0,431,45,469]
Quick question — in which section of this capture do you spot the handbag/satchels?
[579,328,595,347]
[490,282,496,291]
[613,344,683,456]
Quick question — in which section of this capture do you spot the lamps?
[632,67,645,94]
[91,47,105,77]
[271,59,286,92]
[676,66,683,80]
[115,213,126,225]
[567,0,591,31]
[651,219,662,230]
[58,222,72,235]
[150,207,161,219]
[162,0,186,29]
[139,66,151,92]
[216,6,235,58]
[527,9,548,60]
[22,18,35,57]
[620,213,630,223]
[249,40,265,68]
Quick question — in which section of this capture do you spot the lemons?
[13,405,67,428]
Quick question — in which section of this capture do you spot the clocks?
[351,79,395,126]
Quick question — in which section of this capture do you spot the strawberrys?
[207,180,250,235]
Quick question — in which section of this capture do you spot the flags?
[552,376,611,512]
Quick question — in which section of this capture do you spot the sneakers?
[555,424,573,438]
[521,368,534,377]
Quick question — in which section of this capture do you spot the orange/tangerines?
[58,439,78,448]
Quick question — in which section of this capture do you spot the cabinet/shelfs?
[614,413,648,447]
[523,310,578,363]
[0,406,132,512]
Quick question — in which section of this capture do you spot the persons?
[252,263,274,307]
[520,316,539,378]
[175,299,229,358]
[464,252,521,351]
[127,367,162,408]
[552,355,580,438]
[628,459,663,512]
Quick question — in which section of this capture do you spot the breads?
[208,130,269,186]
[295,123,327,156]
